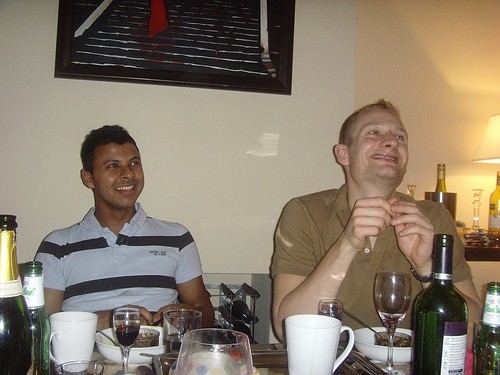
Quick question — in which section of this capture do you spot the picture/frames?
[54,0,296,96]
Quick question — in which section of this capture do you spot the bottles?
[22,261,51,375]
[0,214,31,375]
[435,164,447,192]
[488,171,500,237]
[473,282,500,375]
[412,234,469,375]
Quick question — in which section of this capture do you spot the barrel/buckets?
[425,192,457,222]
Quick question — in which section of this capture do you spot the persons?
[33,124,216,338]
[271,100,481,351]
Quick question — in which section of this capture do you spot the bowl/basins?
[95,325,163,365]
[354,327,412,362]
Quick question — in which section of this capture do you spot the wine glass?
[113,307,141,375]
[373,273,409,375]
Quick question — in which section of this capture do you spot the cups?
[174,328,253,375]
[49,311,99,374]
[374,332,411,347]
[319,300,343,321]
[162,309,203,358]
[284,314,353,375]
[56,360,104,375]
[134,328,160,347]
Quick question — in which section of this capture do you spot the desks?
[463,245,500,261]
[89,340,409,375]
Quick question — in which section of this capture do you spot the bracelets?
[410,268,433,283]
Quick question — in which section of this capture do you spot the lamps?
[472,113,500,163]
